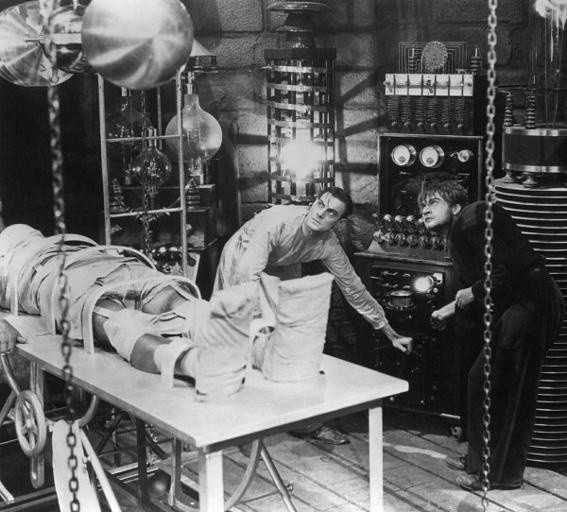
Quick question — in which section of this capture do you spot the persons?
[416,174,562,495]
[216,189,411,446]
[0,220,335,398]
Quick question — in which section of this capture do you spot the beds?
[1,305,411,512]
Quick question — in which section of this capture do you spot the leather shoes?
[446,454,469,469]
[289,423,349,445]
[456,472,524,489]
[240,442,263,460]
